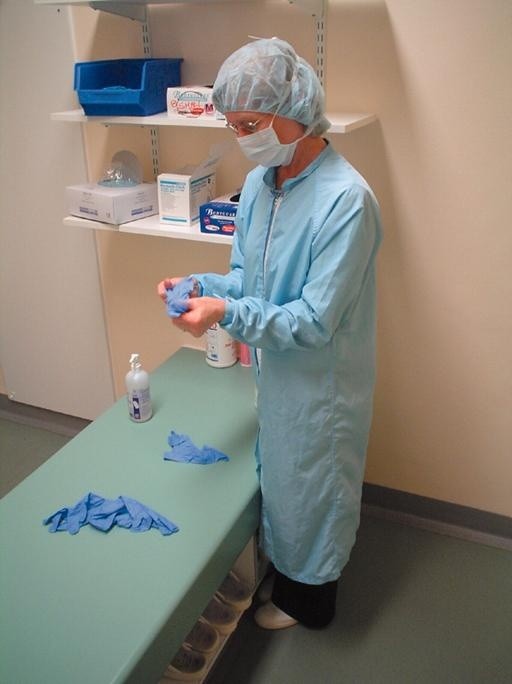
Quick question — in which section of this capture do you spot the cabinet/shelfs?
[47,0,378,246]
[2,346,264,683]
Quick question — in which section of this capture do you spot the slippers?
[163,570,253,681]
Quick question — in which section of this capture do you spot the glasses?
[225,114,269,135]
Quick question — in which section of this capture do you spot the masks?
[235,126,297,168]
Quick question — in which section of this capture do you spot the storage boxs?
[197,189,246,235]
[156,139,229,226]
[65,179,158,227]
[165,81,227,121]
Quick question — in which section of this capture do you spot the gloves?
[162,431,227,465]
[164,280,196,316]
[43,492,179,536]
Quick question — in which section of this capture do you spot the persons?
[158,38,382,635]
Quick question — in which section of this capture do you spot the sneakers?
[254,574,300,630]
